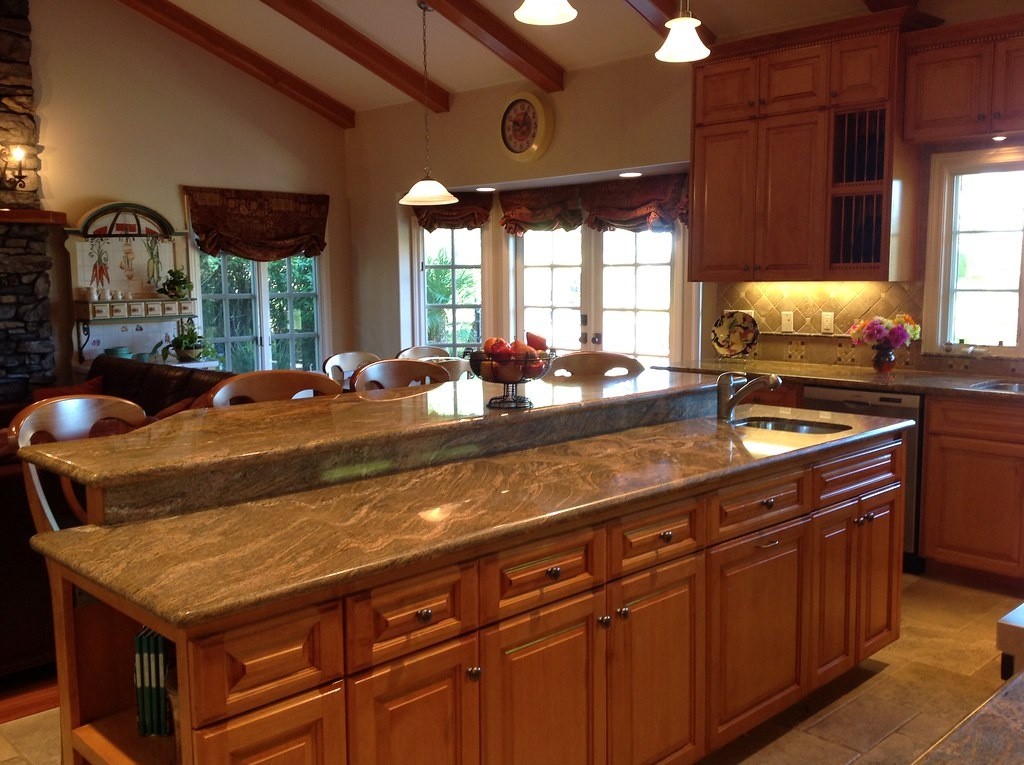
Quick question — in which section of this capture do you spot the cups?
[137,353,149,362]
[123,291,134,299]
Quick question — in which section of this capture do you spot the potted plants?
[159,267,195,301]
[148,315,225,371]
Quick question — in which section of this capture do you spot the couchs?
[0,352,255,679]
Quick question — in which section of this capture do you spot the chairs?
[210,341,645,408]
[6,395,149,538]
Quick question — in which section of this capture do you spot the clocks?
[496,90,557,164]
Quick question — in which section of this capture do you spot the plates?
[710,310,759,358]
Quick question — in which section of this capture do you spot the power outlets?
[821,311,834,334]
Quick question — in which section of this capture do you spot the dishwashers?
[801,387,922,557]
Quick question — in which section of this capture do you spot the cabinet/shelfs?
[75,299,197,365]
[706,453,808,752]
[48,558,480,765]
[692,45,829,126]
[916,392,1024,582]
[808,429,909,710]
[829,31,893,109]
[904,35,1024,154]
[732,379,805,410]
[828,104,920,285]
[477,497,704,765]
[689,111,826,285]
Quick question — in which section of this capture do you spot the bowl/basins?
[106,353,134,359]
[104,346,128,355]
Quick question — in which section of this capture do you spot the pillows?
[33,374,104,402]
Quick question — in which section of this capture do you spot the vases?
[873,343,896,373]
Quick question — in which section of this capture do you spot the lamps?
[0,144,28,192]
[655,0,711,63]
[512,0,579,25]
[399,0,460,206]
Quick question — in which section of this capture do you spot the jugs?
[85,287,125,301]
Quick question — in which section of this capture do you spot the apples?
[481,337,548,382]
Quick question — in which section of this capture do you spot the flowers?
[848,311,923,351]
